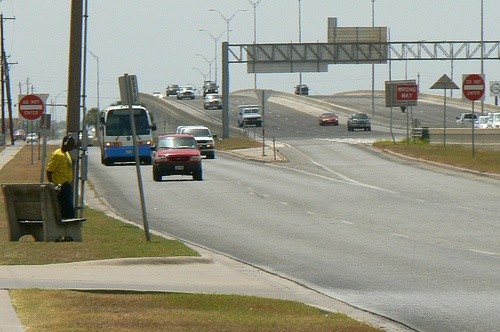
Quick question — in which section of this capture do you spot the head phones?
[64,136,72,146]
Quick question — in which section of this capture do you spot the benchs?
[1,182,86,242]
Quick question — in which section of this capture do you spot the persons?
[46,136,75,219]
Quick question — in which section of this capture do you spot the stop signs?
[463,74,485,101]
[18,95,44,120]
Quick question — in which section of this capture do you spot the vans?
[176,125,217,159]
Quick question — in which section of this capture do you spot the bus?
[99,104,157,166]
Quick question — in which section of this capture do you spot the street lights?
[197,28,233,85]
[195,53,222,81]
[209,8,248,44]
[81,46,101,147]
[192,66,218,82]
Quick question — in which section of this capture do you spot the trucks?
[236,104,264,128]
[294,84,309,95]
[202,80,219,97]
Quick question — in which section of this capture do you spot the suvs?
[347,113,372,131]
[166,84,180,97]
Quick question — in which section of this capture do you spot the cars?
[203,94,222,110]
[150,134,208,181]
[79,132,94,146]
[473,112,500,130]
[26,132,38,144]
[153,91,162,99]
[318,113,339,126]
[176,86,197,100]
[13,129,27,141]
[455,113,479,128]
